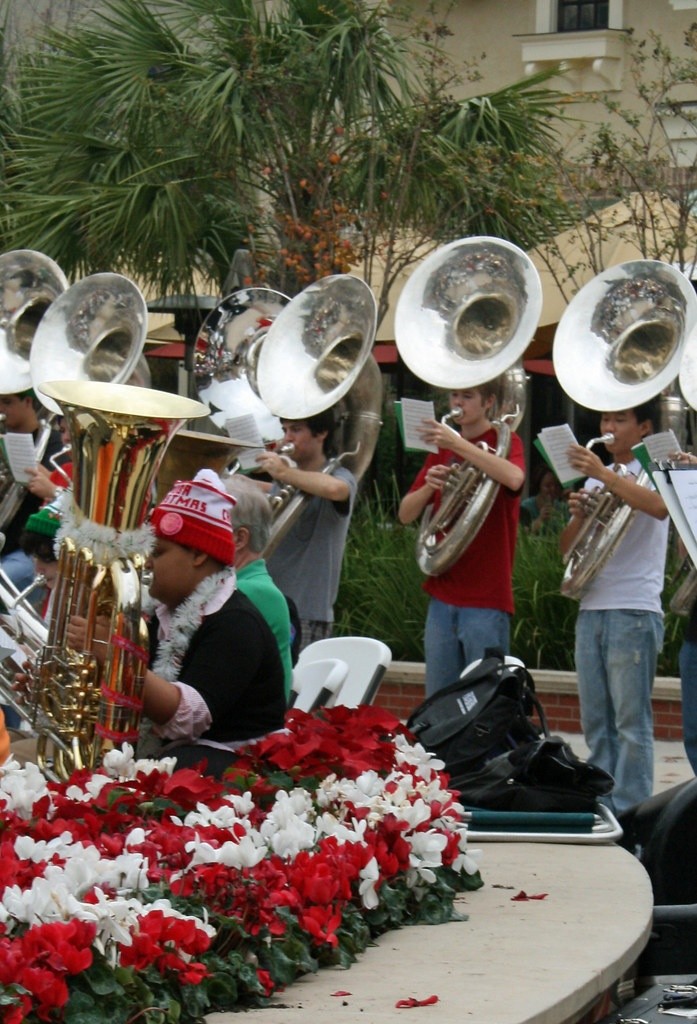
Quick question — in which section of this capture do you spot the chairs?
[288,637,391,715]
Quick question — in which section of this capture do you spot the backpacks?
[447,735,617,812]
[405,647,552,776]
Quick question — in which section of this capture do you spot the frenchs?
[0,249,70,394]
[394,234,543,579]
[27,271,147,492]
[551,258,697,598]
[255,272,386,560]
[187,287,293,475]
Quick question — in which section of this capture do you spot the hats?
[144,468,239,567]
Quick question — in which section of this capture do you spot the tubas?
[0,379,212,803]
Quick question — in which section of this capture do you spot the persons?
[400,386,527,701]
[667,397,697,780]
[251,414,357,657]
[1,388,291,762]
[519,465,571,535]
[557,403,671,816]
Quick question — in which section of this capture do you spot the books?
[532,423,590,488]
[631,429,686,495]
[224,413,266,474]
[0,433,37,484]
[393,398,438,454]
